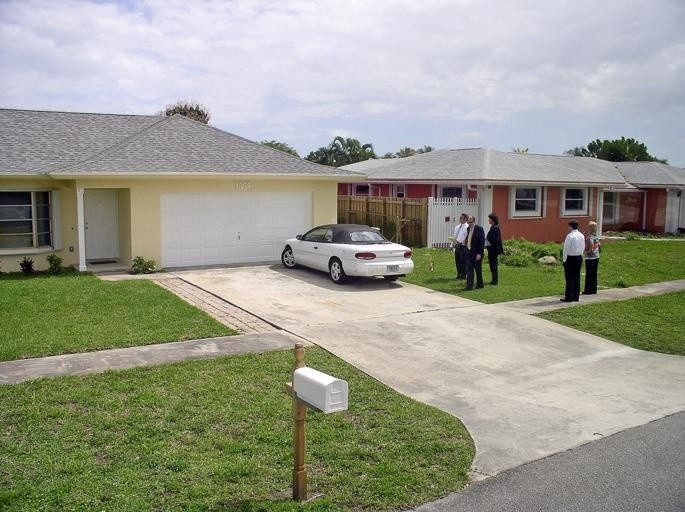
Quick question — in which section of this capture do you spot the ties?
[455,224,462,239]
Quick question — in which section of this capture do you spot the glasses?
[467,221,474,224]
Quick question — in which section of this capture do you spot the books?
[448,237,459,245]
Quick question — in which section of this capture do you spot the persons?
[449,213,470,280]
[583,220,601,295]
[560,219,586,302]
[484,213,505,285]
[457,215,486,291]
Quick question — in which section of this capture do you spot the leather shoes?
[455,277,460,280]
[560,299,567,302]
[461,277,466,281]
[582,291,590,294]
[572,298,579,301]
[591,291,597,294]
[475,286,484,289]
[464,287,473,291]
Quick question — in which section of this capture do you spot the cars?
[281,224,414,284]
